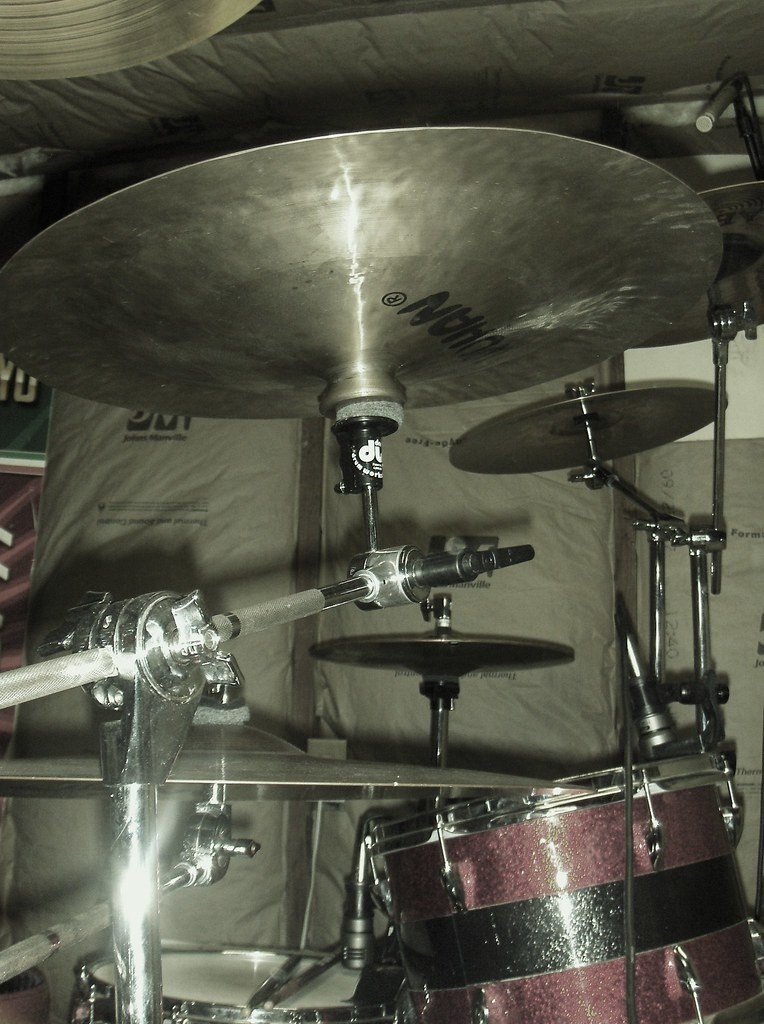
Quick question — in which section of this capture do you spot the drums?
[72,944,403,1024]
[368,749,762,1022]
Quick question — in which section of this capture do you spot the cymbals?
[622,182,764,349]
[0,1,266,82]
[447,378,730,474]
[0,126,724,420]
[1,724,590,786]
[308,628,578,675]
